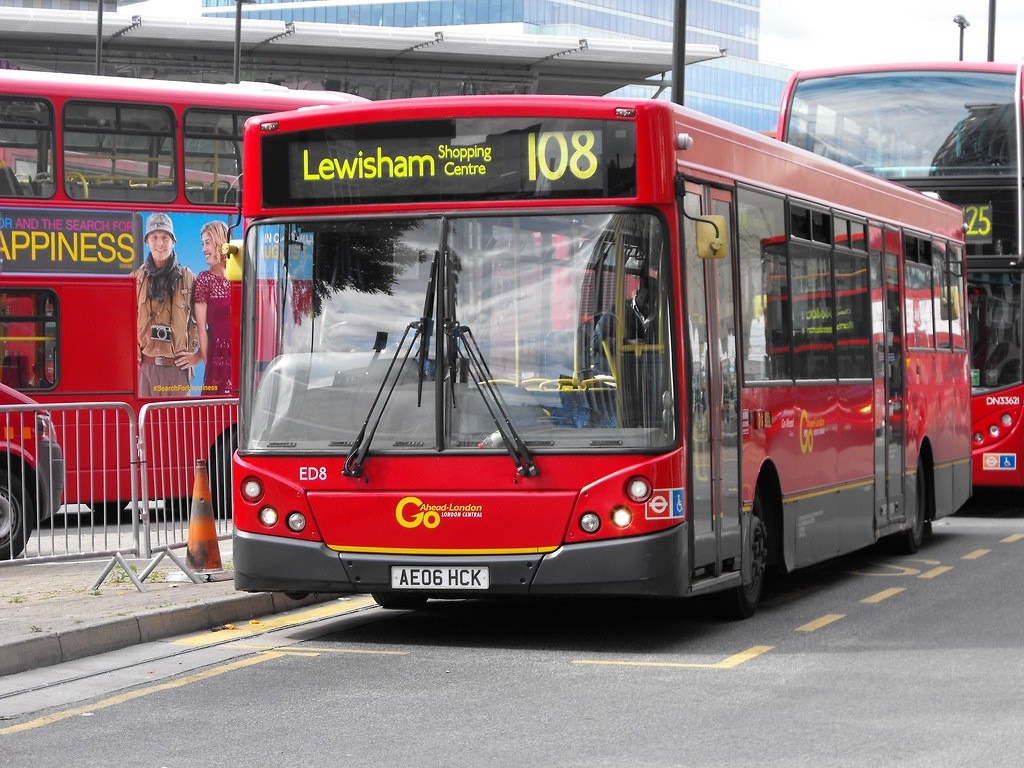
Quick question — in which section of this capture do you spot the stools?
[540,330,575,379]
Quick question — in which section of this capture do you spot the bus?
[757,230,962,379]
[229,92,973,617]
[0,63,375,562]
[777,62,1023,518]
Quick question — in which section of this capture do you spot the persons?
[590,275,660,429]
[128,213,202,397]
[193,220,232,396]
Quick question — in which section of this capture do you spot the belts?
[142,354,176,366]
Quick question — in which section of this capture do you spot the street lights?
[953,13,970,62]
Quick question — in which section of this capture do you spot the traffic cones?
[185,457,232,582]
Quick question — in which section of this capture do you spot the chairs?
[1,164,240,205]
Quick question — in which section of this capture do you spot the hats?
[144,213,177,242]
